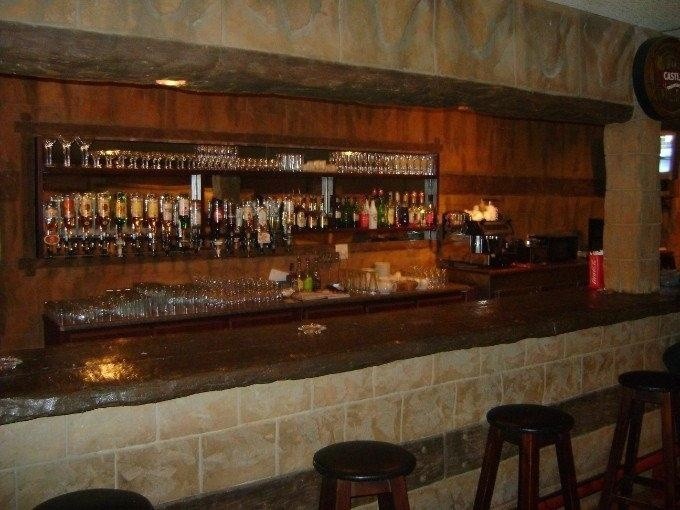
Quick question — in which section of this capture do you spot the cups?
[43,276,288,325]
[327,257,446,297]
[238,153,435,173]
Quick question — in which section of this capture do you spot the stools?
[471,403,582,510]
[312,439,416,510]
[596,369,680,510]
[31,487,156,510]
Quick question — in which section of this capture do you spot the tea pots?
[467,200,498,221]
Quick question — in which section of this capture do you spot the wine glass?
[45,136,238,170]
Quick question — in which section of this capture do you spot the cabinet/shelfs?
[13,120,445,277]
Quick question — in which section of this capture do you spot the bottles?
[44,188,437,261]
[288,254,322,293]
